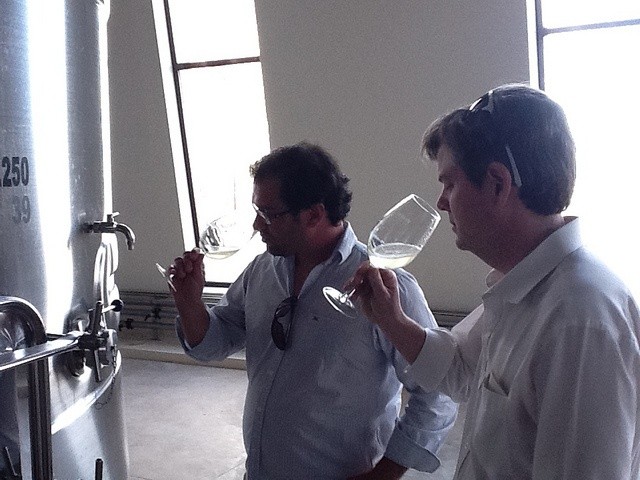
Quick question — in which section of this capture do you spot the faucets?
[88,211,135,251]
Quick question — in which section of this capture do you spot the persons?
[342,83,638,479]
[168,140,461,479]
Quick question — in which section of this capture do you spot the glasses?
[271,295,298,350]
[468,88,523,189]
[252,192,291,225]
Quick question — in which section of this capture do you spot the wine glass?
[155,210,260,294]
[322,193,441,319]
